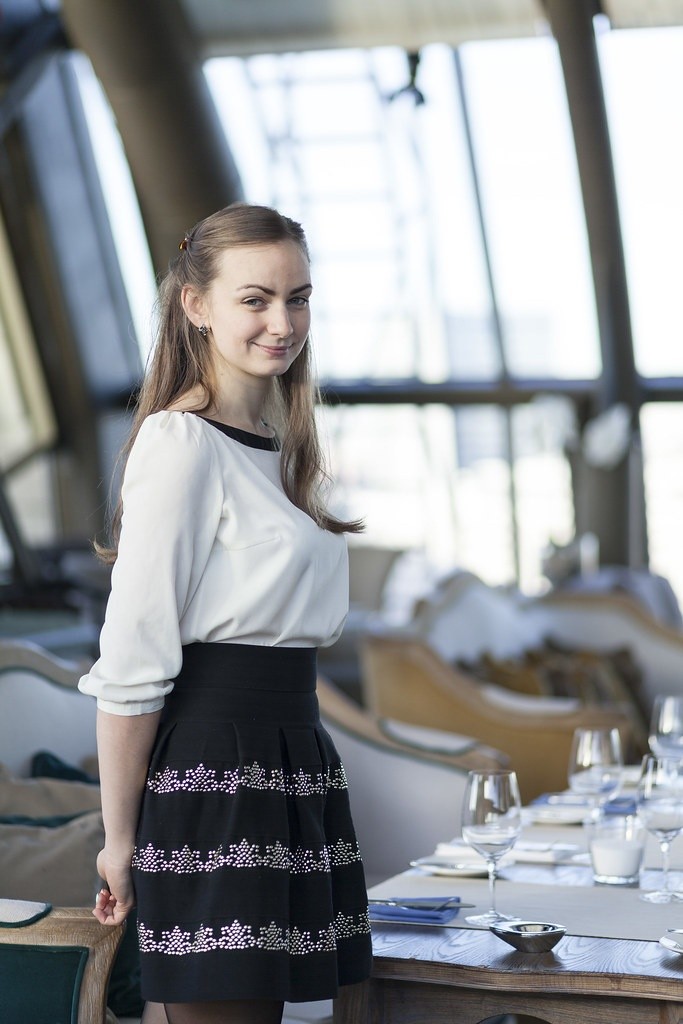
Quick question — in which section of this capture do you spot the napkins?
[529,792,640,814]
[369,896,460,924]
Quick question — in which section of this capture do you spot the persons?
[78,206,371,1024]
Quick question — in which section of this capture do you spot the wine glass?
[635,754,683,905]
[461,768,523,927]
[567,726,624,821]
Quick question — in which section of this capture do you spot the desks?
[331,765,683,1024]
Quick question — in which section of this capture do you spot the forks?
[517,839,558,852]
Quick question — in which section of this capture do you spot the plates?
[660,929,683,953]
[614,763,645,785]
[522,806,589,824]
[417,855,496,876]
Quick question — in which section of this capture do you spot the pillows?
[0,768,106,908]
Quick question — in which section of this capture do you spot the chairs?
[0,635,511,1024]
[356,564,683,805]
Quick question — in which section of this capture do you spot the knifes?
[368,900,476,907]
[410,860,489,870]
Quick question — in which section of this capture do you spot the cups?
[648,693,683,769]
[582,815,647,885]
